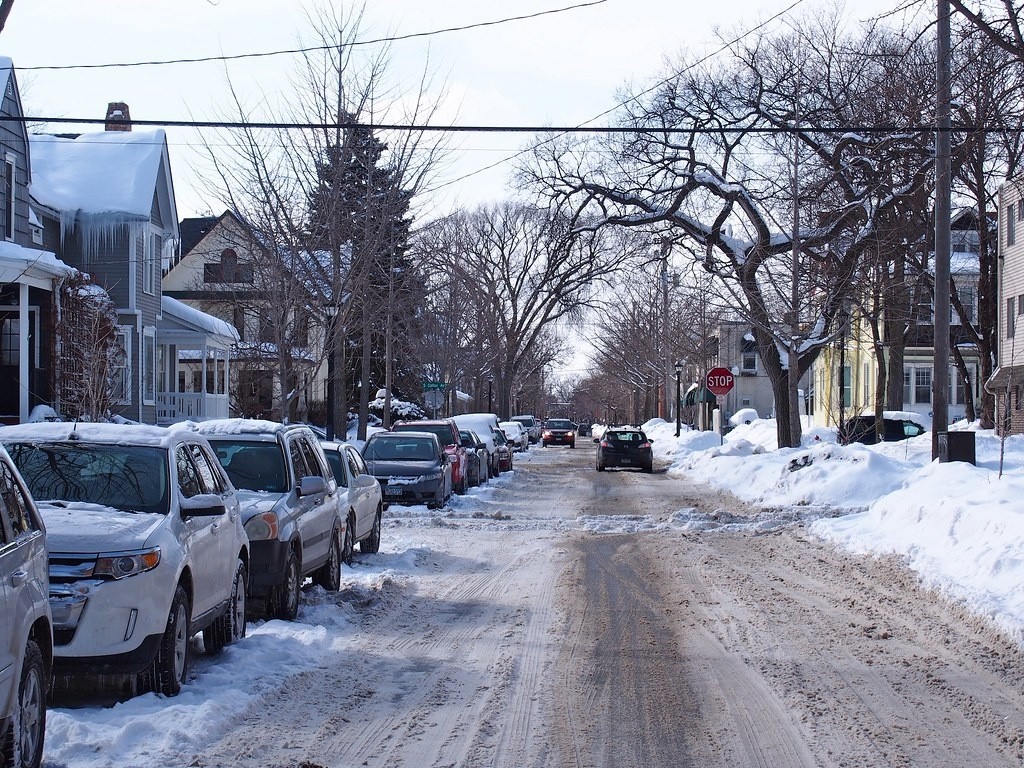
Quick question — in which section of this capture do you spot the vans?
[836,414,926,446]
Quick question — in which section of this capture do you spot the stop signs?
[706,367,735,397]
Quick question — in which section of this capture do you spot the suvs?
[0,421,251,698]
[388,418,469,495]
[535,418,579,449]
[0,441,53,768]
[510,415,539,445]
[167,424,348,619]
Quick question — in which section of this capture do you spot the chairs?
[416,443,431,457]
[122,452,158,496]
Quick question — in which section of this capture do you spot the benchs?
[610,436,639,439]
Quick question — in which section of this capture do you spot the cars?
[318,440,384,568]
[496,421,529,453]
[593,427,654,474]
[577,423,593,438]
[357,430,453,511]
[440,427,489,488]
[484,437,499,479]
[492,428,514,471]
